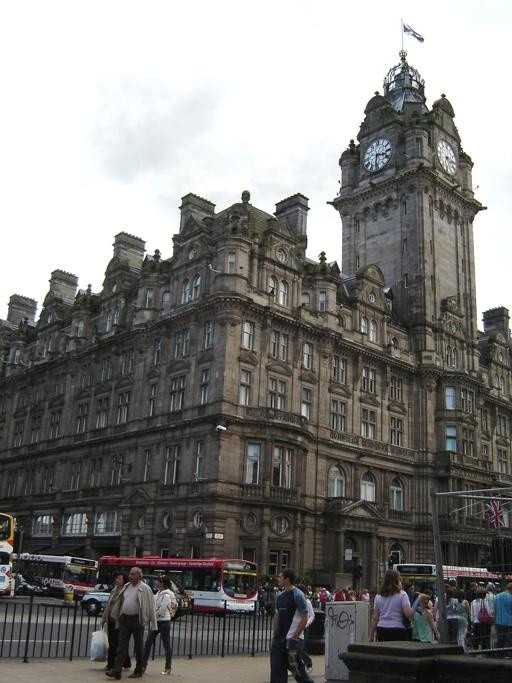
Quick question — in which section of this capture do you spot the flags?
[402,22,425,46]
[486,500,506,530]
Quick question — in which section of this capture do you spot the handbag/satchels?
[478,597,492,624]
[90,624,109,662]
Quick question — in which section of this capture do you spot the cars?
[13,572,50,596]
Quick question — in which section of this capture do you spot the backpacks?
[162,593,179,617]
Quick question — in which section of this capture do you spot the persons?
[286,584,315,676]
[269,568,315,683]
[367,569,426,643]
[105,567,159,681]
[259,582,370,618]
[144,573,174,674]
[99,573,133,673]
[401,576,511,661]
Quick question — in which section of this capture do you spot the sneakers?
[305,667,313,674]
[103,666,142,680]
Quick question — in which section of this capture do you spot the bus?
[391,561,492,590]
[0,510,16,596]
[96,552,262,616]
[10,550,101,598]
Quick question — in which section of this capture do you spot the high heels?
[160,668,171,675]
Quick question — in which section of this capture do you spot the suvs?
[79,572,193,623]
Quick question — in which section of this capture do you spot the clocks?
[362,136,393,173]
[436,139,458,176]
